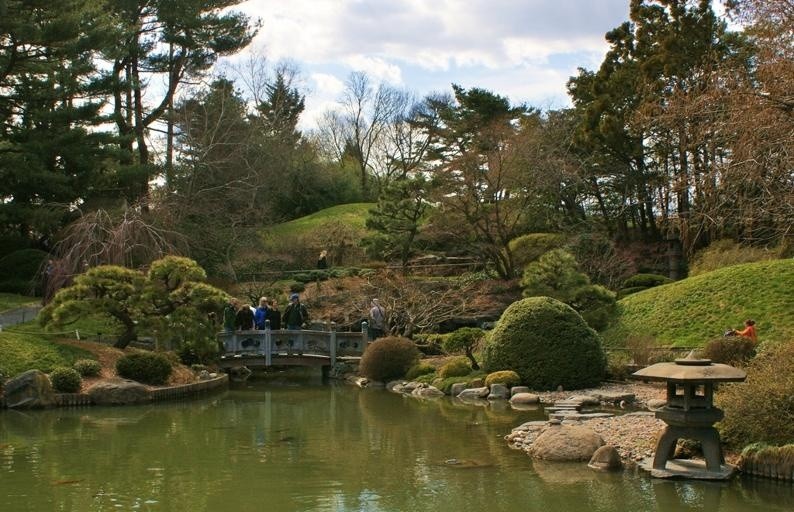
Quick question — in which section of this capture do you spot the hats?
[288,293,299,300]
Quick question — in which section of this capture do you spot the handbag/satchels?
[301,320,312,329]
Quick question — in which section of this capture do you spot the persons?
[724,318,757,347]
[317,248,330,270]
[369,298,386,342]
[223,296,281,358]
[281,293,308,356]
